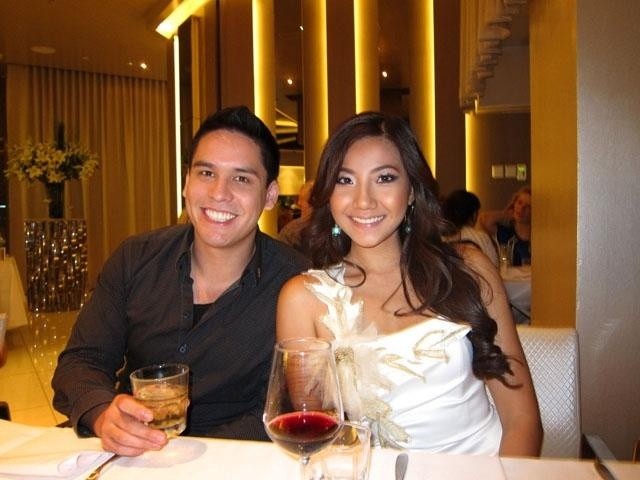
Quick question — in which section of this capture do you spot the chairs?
[515,324,619,460]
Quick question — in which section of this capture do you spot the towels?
[0,419,116,478]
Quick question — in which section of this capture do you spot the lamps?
[152,0,211,42]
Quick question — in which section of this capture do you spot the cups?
[128,362,191,438]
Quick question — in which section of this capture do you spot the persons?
[279,182,326,266]
[276,111,544,458]
[52,107,315,458]
[439,188,532,268]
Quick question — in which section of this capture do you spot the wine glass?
[261,337,345,480]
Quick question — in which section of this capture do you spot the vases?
[45,182,66,219]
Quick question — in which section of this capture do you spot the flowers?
[0,120,101,184]
